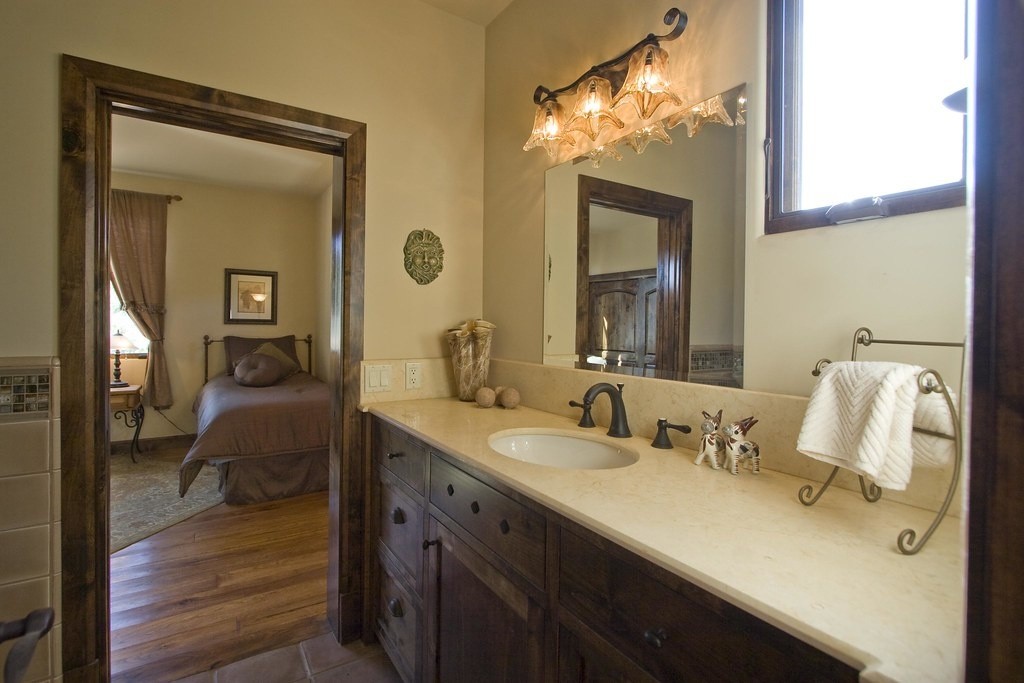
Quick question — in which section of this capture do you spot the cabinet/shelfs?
[372,415,861,683]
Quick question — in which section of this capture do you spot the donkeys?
[694,409,760,475]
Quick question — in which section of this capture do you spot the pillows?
[223,336,302,376]
[232,342,300,383]
[234,355,282,388]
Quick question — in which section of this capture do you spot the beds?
[179,334,331,506]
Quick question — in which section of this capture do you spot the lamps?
[523,7,687,161]
[249,293,268,317]
[579,89,746,168]
[110,329,139,388]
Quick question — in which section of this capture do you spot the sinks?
[486,426,641,471]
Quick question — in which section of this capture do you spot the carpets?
[110,447,226,556]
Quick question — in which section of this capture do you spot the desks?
[110,385,144,463]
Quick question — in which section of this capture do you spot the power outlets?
[404,362,422,390]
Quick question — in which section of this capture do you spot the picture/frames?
[223,268,278,325]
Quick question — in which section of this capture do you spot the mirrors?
[545,82,747,392]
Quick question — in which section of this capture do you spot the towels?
[793,359,960,494]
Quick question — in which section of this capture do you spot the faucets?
[583,382,633,439]
